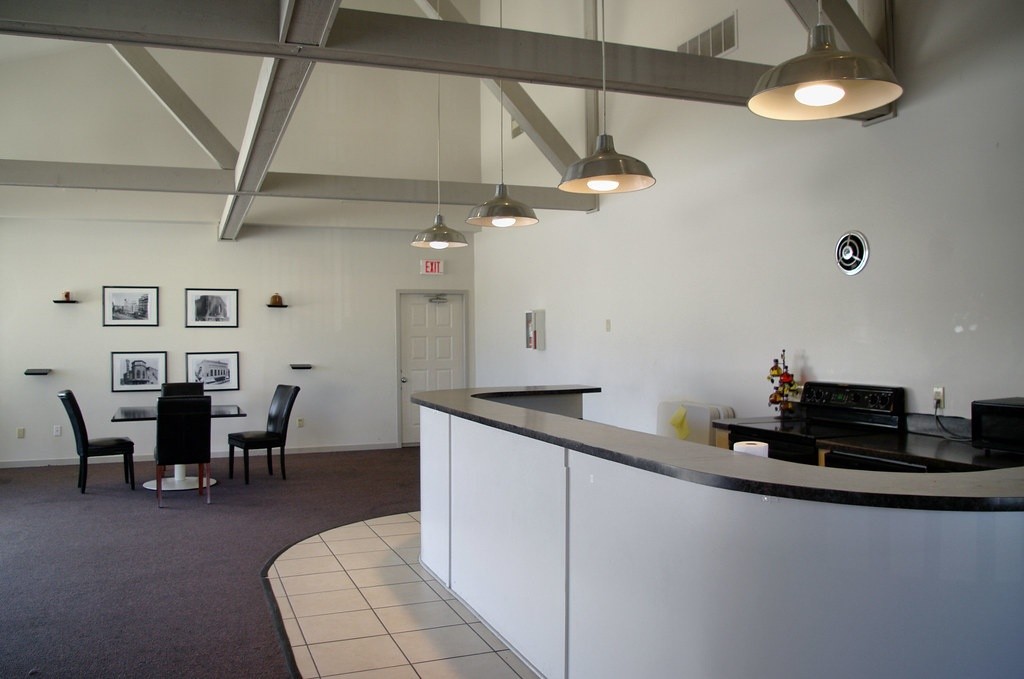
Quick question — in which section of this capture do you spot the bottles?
[270,293,282,304]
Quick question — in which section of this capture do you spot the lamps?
[465,0,539,229]
[746,0,905,122]
[409,0,468,249]
[557,0,656,196]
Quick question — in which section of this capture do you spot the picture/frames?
[101,284,159,326]
[110,351,168,392]
[185,351,241,391]
[184,287,239,328]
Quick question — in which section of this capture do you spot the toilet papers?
[734,441,768,458]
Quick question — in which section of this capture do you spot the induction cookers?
[733,381,906,442]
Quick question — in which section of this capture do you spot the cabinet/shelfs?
[710,410,1023,476]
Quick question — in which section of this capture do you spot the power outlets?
[933,386,944,409]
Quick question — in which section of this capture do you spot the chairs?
[56,390,136,495]
[152,396,212,507]
[227,384,300,486]
[157,381,206,469]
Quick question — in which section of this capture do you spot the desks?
[110,404,248,491]
